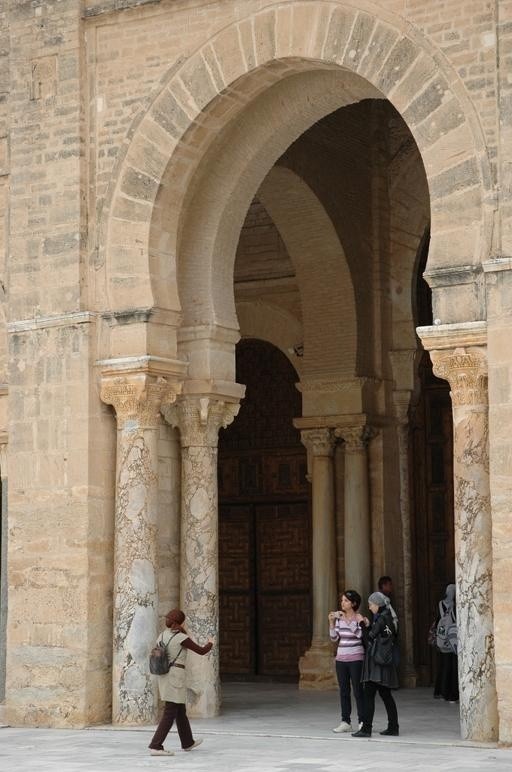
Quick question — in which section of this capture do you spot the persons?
[327,590,367,733]
[433,584,458,705]
[351,591,403,737]
[377,576,402,689]
[148,609,215,756]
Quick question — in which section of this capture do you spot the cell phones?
[332,612,341,619]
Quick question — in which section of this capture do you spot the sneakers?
[184,738,203,751]
[332,720,352,733]
[380,727,400,736]
[148,748,175,756]
[352,729,371,737]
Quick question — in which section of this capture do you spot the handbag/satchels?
[368,616,396,667]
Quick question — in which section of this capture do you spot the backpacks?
[149,631,185,675]
[435,601,456,653]
